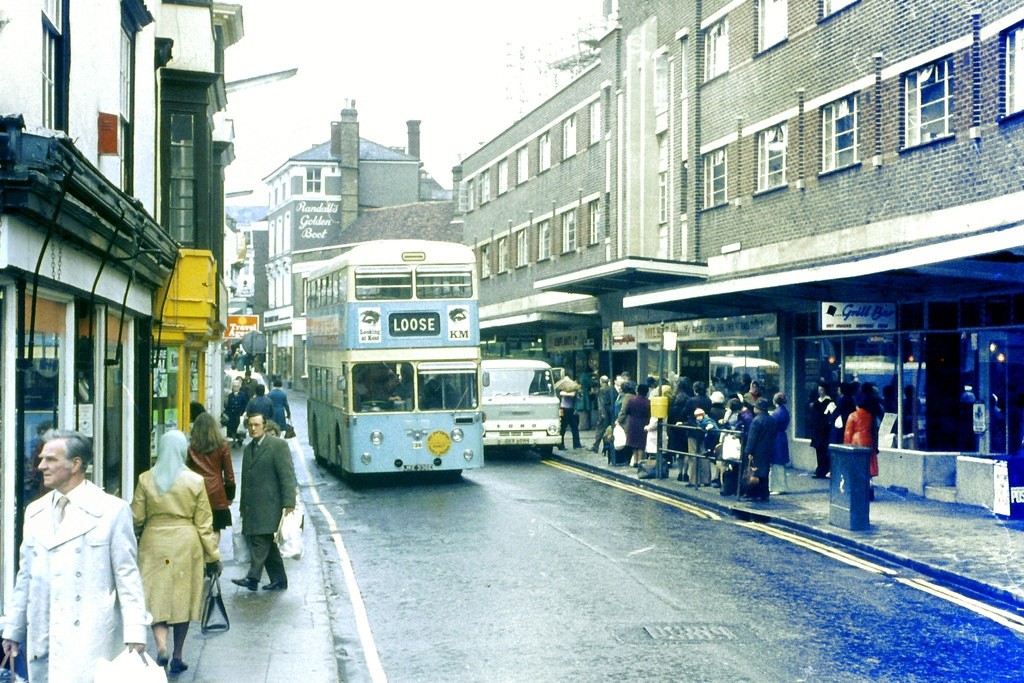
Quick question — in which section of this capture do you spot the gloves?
[206,561,222,577]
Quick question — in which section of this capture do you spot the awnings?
[533,255,706,296]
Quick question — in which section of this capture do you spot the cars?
[223,371,269,408]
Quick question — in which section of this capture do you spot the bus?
[843,360,926,440]
[304,239,490,486]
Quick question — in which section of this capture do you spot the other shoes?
[812,473,827,478]
[232,444,236,448]
[586,448,598,453]
[558,446,585,453]
[677,474,720,489]
[770,491,781,495]
[634,463,638,468]
[157,650,187,673]
[869,489,874,500]
[721,491,769,501]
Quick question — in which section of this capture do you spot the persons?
[422,374,455,409]
[376,373,403,401]
[2,429,152,683]
[844,396,880,477]
[185,412,236,546]
[830,382,852,447]
[559,369,789,500]
[843,382,920,435]
[230,412,296,590]
[223,370,289,448]
[130,429,222,672]
[30,421,55,495]
[809,384,837,479]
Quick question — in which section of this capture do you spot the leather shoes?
[231,577,287,591]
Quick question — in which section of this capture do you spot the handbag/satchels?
[236,416,248,434]
[746,457,760,485]
[284,417,296,439]
[0,651,27,683]
[638,455,669,479]
[723,435,741,460]
[613,422,626,451]
[201,573,229,634]
[93,646,168,683]
[221,410,231,426]
[275,508,304,560]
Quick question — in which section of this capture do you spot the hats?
[752,391,787,411]
[694,408,704,414]
[710,392,723,403]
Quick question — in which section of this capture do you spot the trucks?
[710,356,780,381]
[477,359,565,460]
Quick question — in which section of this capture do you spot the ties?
[252,441,259,459]
[55,497,69,523]
